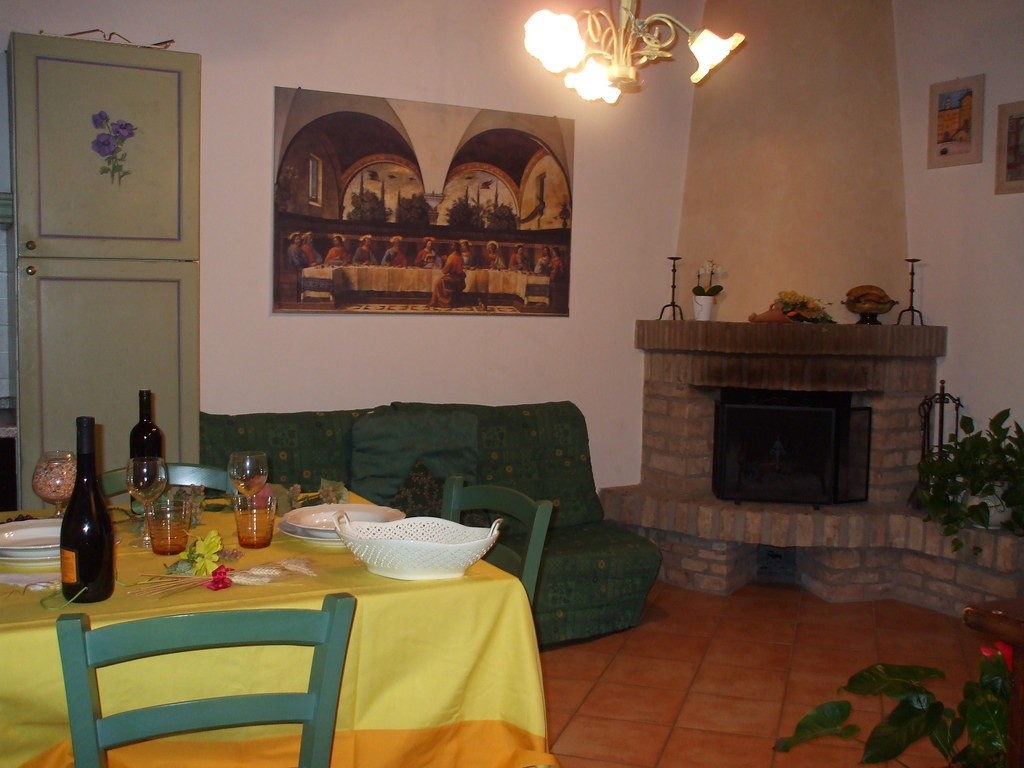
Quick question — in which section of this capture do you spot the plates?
[0,517,66,557]
[276,503,407,545]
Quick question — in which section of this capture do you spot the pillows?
[390,449,490,527]
[348,413,485,511]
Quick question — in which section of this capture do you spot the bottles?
[61,416,115,603]
[130,389,164,498]
[143,500,193,556]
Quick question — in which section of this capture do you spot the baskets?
[331,510,503,580]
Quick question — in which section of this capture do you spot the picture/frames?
[995,101,1024,195]
[924,72,986,170]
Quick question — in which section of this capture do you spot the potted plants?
[915,409,1023,555]
[688,262,721,320]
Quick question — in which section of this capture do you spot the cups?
[232,495,277,549]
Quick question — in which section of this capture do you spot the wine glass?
[230,450,269,516]
[127,459,168,550]
[32,450,77,519]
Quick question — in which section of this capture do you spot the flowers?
[773,638,1015,768]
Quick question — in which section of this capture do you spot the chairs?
[54,589,357,768]
[442,476,554,609]
[98,463,237,512]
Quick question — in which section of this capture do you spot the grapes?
[184,484,205,523]
[287,483,338,504]
[218,548,241,560]
[776,289,814,304]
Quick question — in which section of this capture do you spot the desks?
[1,486,555,767]
[302,264,551,308]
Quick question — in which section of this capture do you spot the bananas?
[847,285,890,304]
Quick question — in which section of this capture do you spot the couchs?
[188,401,660,654]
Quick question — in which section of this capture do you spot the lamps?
[522,0,745,105]
